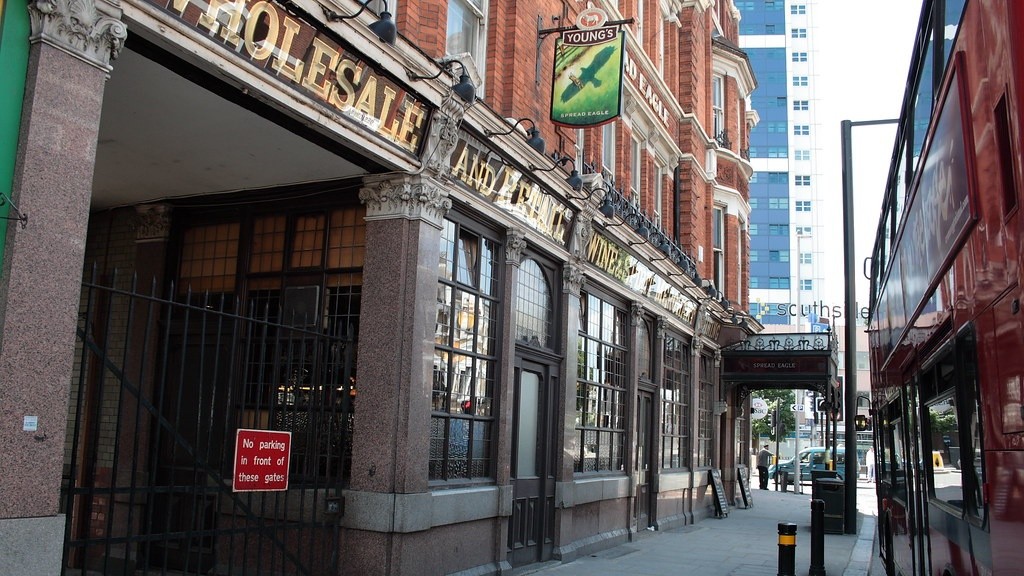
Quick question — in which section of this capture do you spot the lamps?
[683,278,718,297]
[724,312,748,329]
[602,214,649,239]
[566,187,614,221]
[696,291,730,308]
[628,232,671,257]
[530,157,583,192]
[648,248,689,271]
[712,307,735,324]
[410,60,475,106]
[485,118,545,157]
[668,266,703,288]
[327,0,397,47]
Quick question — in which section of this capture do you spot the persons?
[757,445,773,490]
[866,446,876,483]
[802,454,819,463]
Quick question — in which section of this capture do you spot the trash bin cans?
[882,477,905,505]
[815,478,844,535]
[810,470,838,501]
[949,446,960,465]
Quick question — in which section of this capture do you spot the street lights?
[795,234,813,494]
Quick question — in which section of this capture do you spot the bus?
[854,1,1024,576]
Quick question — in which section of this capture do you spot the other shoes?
[873,481,876,483]
[867,480,871,483]
[760,487,769,490]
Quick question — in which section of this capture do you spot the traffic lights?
[816,398,827,411]
[767,411,776,427]
[830,377,843,421]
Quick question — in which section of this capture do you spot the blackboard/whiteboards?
[709,469,729,514]
[738,467,753,506]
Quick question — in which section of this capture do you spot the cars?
[857,445,913,474]
[768,447,860,485]
